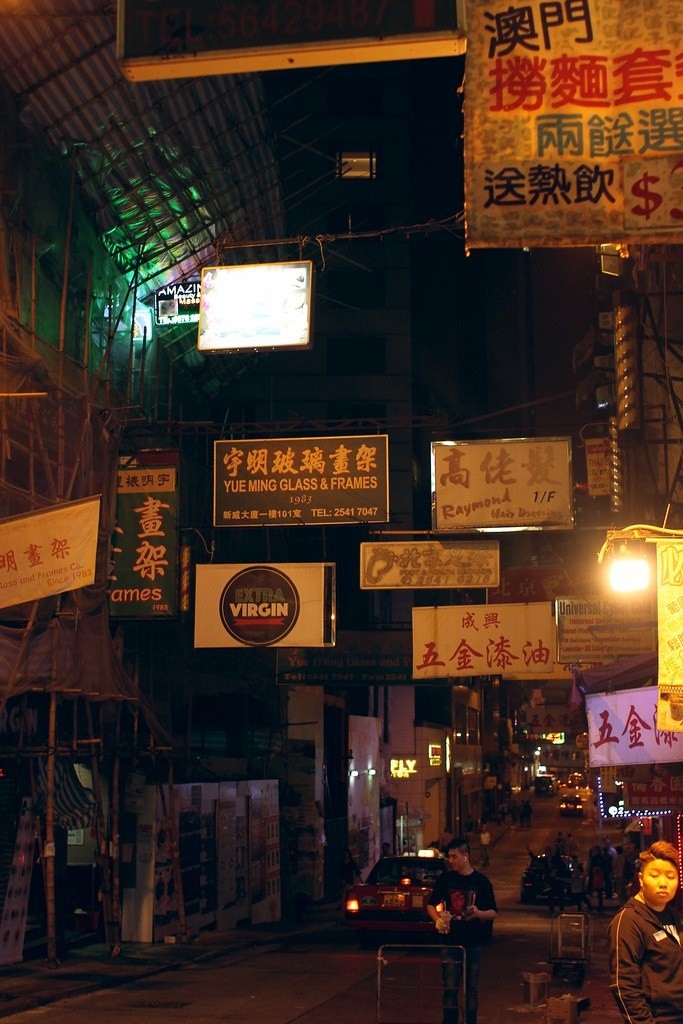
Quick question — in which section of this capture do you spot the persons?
[378,843,394,857]
[606,840,683,1024]
[526,842,639,915]
[500,799,532,831]
[554,832,581,864]
[479,825,492,869]
[335,853,358,910]
[424,836,498,1024]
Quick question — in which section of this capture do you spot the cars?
[346,857,453,940]
[521,855,577,903]
[560,795,583,817]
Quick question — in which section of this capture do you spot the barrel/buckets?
[520,970,552,1009]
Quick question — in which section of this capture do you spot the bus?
[535,774,560,797]
